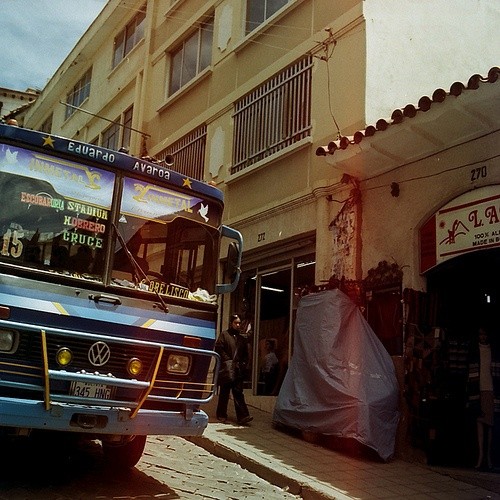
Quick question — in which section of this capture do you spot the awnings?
[313,67,499,182]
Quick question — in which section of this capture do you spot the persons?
[256,339,286,396]
[215,314,254,424]
[405,321,499,473]
[19,217,150,285]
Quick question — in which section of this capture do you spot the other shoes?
[237,415,254,425]
[217,416,233,424]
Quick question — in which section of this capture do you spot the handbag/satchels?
[237,363,250,378]
[216,360,234,387]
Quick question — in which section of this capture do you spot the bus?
[1,119,244,476]
